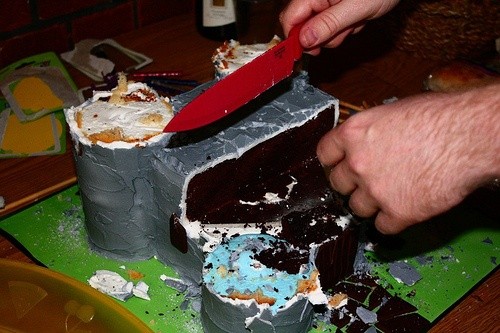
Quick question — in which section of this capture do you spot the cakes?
[60,35,434,333]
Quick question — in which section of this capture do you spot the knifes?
[162,21,312,134]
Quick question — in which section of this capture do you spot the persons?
[277,0,500,234]
